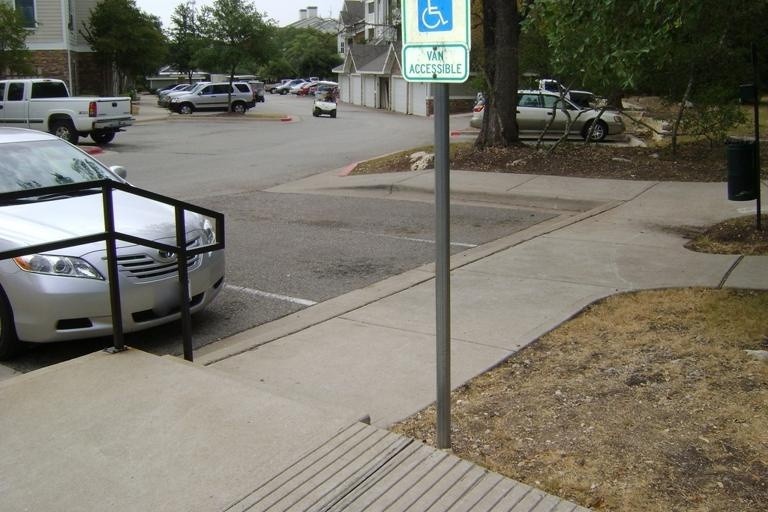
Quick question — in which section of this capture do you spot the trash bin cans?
[727,144,757,201]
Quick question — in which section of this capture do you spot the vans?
[470,90,626,141]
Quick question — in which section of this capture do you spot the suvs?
[156,79,255,114]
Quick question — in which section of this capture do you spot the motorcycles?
[248,82,265,102]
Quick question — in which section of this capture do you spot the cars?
[264,79,338,95]
[0,127,226,363]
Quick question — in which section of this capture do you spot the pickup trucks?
[0,78,132,145]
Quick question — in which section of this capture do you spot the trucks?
[480,78,571,103]
